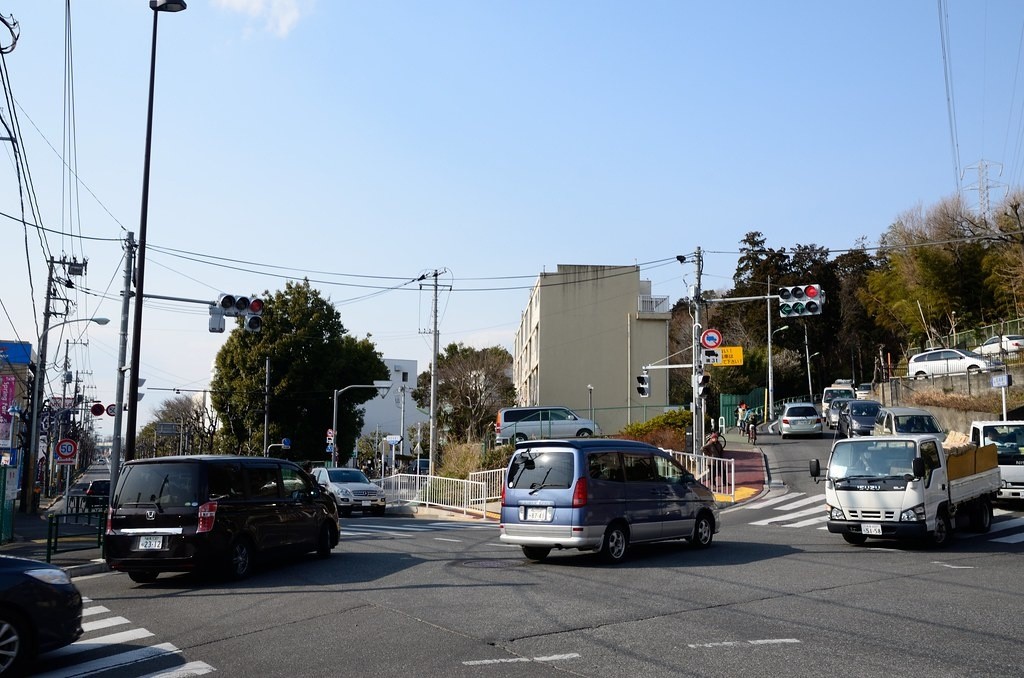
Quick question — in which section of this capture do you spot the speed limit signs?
[107,403,116,416]
[56,440,76,459]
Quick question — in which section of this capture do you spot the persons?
[700,434,732,487]
[299,461,328,494]
[737,404,758,434]
[855,446,873,472]
[984,428,1003,449]
[911,418,928,432]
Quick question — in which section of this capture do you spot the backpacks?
[748,412,758,422]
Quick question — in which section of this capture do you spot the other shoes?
[710,483,719,487]
[723,482,731,486]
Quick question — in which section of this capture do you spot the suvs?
[310,466,387,517]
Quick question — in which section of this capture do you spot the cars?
[1,554,84,676]
[409,459,431,485]
[779,335,1024,447]
[84,480,111,507]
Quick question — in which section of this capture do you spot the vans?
[497,406,601,447]
[497,438,718,558]
[101,456,341,585]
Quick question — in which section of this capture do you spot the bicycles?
[707,418,757,447]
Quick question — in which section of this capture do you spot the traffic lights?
[128,377,146,403]
[697,374,711,397]
[220,295,264,333]
[780,284,823,317]
[637,373,651,399]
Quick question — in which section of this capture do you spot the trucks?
[947,421,1024,501]
[809,437,1003,549]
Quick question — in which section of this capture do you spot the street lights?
[22,1,187,541]
[766,325,820,421]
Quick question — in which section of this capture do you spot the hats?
[51,483,56,486]
[711,434,718,438]
